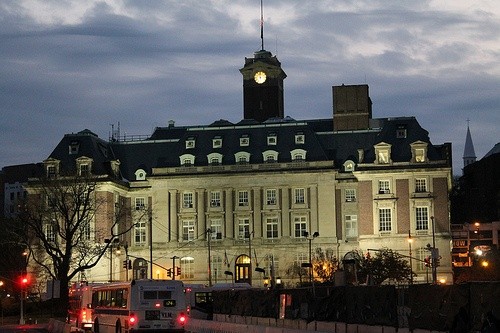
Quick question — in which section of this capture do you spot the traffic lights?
[123,259,127,268]
[167,267,172,277]
[436,258,441,269]
[177,266,182,276]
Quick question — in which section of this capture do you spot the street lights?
[103,238,120,282]
[407,229,413,285]
[19,279,28,325]
[302,231,320,285]
[473,222,481,245]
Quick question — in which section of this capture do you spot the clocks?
[254,71,266,84]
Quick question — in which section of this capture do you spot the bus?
[65,282,102,328]
[80,278,187,333]
